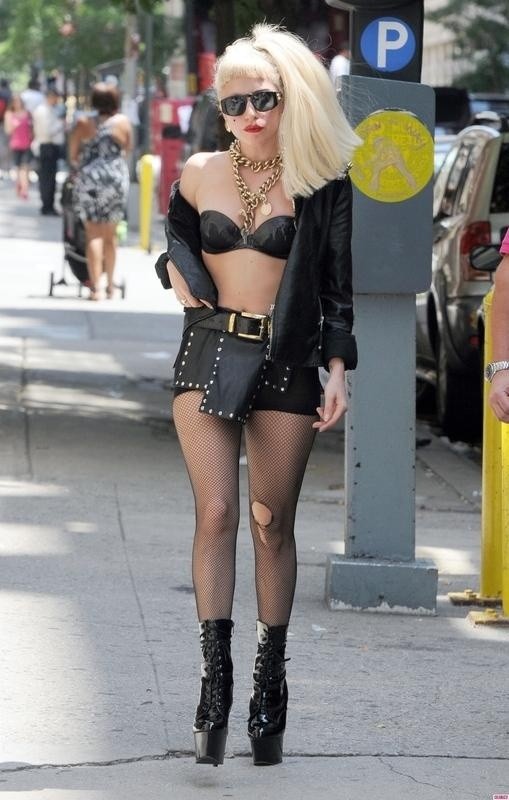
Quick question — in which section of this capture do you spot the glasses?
[219,91,283,116]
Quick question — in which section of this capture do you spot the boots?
[247,619,288,765]
[193,618,234,767]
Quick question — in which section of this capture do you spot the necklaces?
[230,138,284,245]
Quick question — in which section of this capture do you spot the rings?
[181,299,187,305]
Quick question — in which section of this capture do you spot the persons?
[155,23,365,766]
[67,81,134,301]
[329,42,352,87]
[490,227,509,423]
[0,75,69,216]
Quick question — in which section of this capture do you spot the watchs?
[485,360,509,383]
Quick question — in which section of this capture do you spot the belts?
[192,309,273,342]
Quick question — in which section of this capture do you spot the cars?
[417,91,509,441]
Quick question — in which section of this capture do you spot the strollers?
[49,173,125,298]
